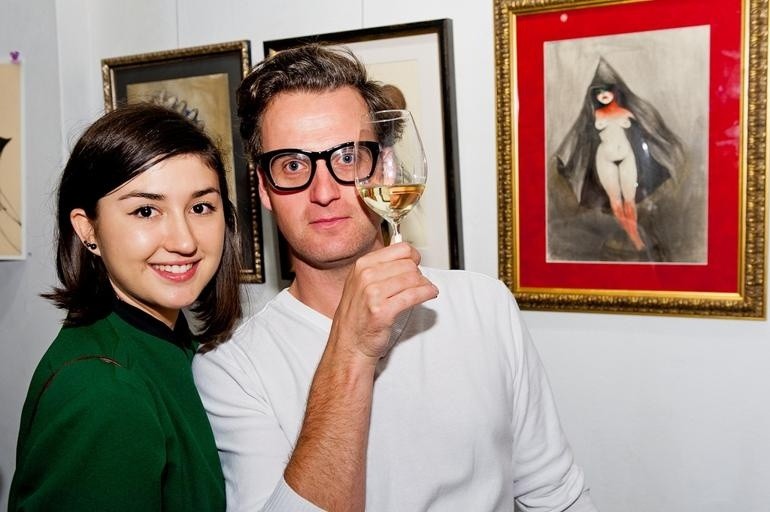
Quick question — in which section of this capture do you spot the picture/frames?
[99,39,265,284]
[492,2,764,321]
[262,16,465,293]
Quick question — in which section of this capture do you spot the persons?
[192,44,596,511]
[7,100,244,512]
[556,55,677,255]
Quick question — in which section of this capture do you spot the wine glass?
[354,108,428,247]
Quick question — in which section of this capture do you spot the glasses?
[256,140,379,192]
